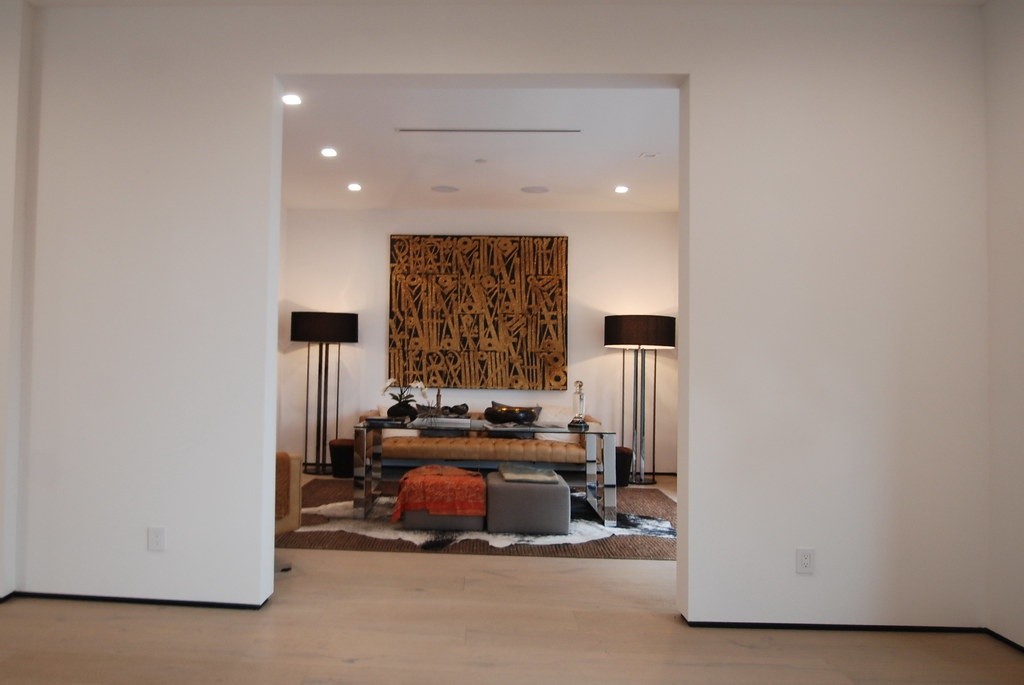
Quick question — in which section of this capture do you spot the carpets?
[275,478,677,561]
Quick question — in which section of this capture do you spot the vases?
[387,402,418,421]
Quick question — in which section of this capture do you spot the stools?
[484,470,570,536]
[601,445,633,487]
[275,452,302,537]
[401,464,486,531]
[329,438,354,478]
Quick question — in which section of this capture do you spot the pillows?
[417,403,470,437]
[486,400,542,439]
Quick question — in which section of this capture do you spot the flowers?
[383,376,425,403]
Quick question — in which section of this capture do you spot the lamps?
[290,311,359,477]
[603,314,676,484]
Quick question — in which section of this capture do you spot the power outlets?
[796,546,814,573]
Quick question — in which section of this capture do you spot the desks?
[351,414,620,528]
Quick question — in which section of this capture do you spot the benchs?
[359,403,605,472]
